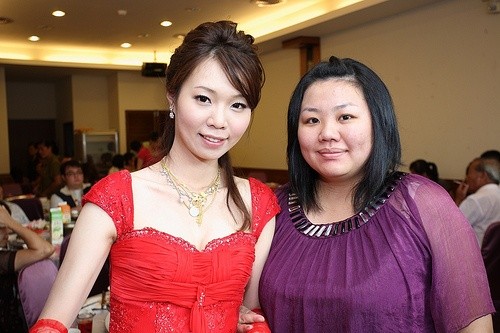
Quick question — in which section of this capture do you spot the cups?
[76,311,97,333]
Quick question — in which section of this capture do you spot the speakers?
[141,63,167,77]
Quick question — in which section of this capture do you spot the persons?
[0,202,56,333]
[409,149,500,250]
[27,19,281,333]
[236,54,495,333]
[0,123,166,297]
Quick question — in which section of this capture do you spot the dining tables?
[7,217,78,251]
[68,294,110,333]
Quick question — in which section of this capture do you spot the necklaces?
[157,156,224,225]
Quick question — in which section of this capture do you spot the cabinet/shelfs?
[75,129,118,160]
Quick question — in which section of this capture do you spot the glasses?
[65,169,83,177]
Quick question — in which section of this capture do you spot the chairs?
[7,197,45,225]
[480,222,500,304]
[18,258,58,325]
